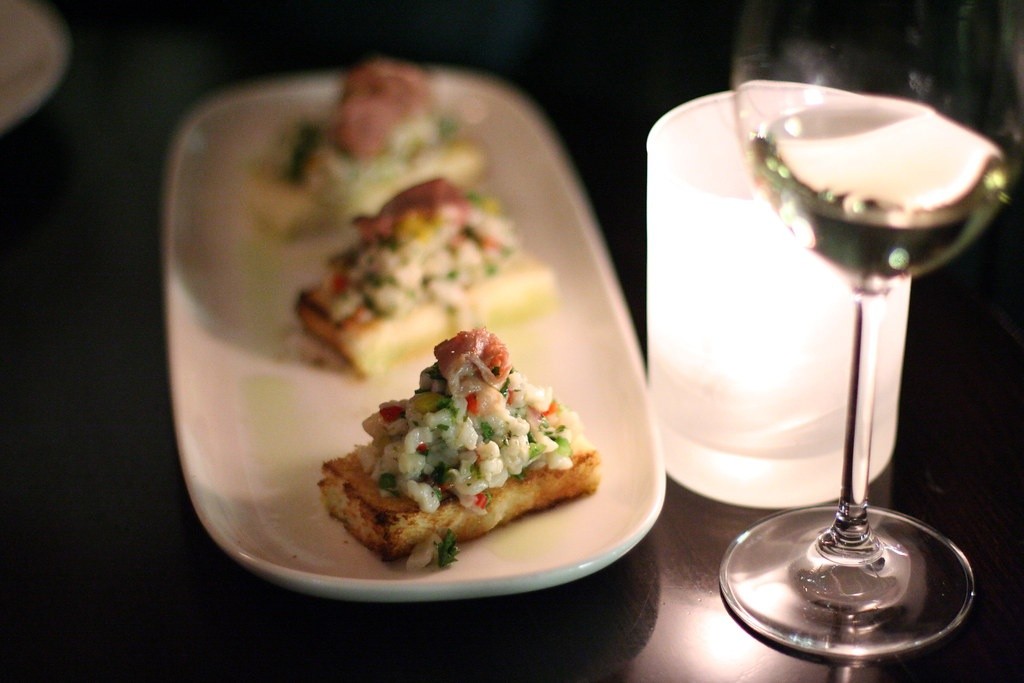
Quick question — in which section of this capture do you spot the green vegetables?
[348,197,567,568]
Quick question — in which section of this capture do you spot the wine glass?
[720,0,1024,656]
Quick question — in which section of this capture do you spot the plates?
[1,0,70,138]
[160,67,668,603]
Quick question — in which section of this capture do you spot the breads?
[293,264,560,378]
[318,446,599,561]
[279,129,480,210]
